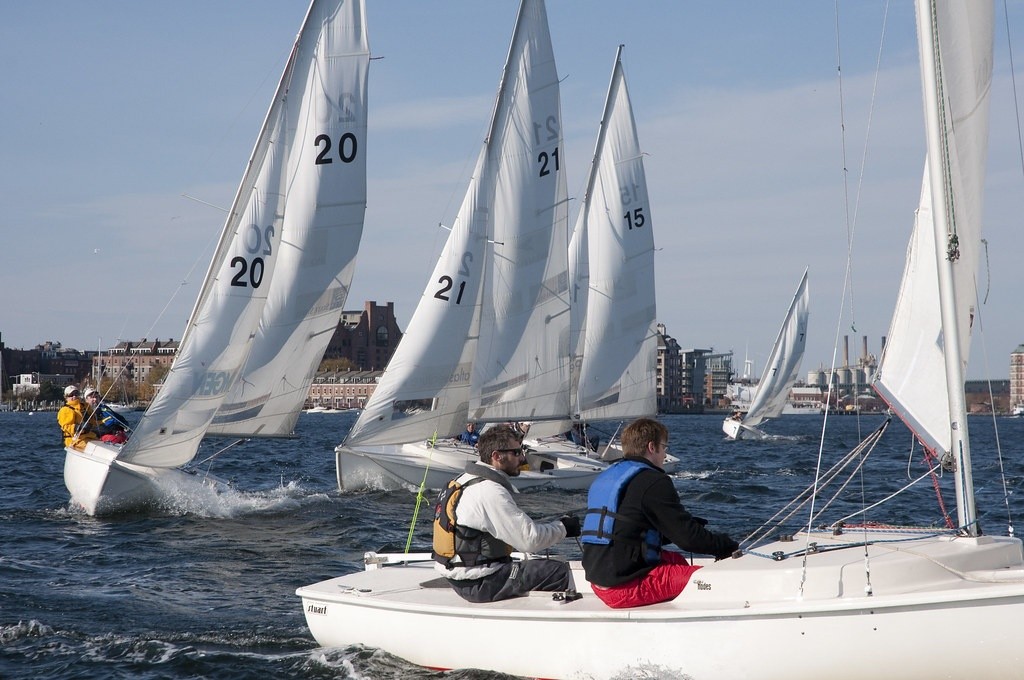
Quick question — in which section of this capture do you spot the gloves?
[75,422,93,434]
[691,517,709,528]
[716,532,739,562]
[560,516,581,537]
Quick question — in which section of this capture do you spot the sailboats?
[60,2,1024,677]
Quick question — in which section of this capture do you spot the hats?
[63,384,79,397]
[84,388,99,399]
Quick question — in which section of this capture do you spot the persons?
[431,424,583,604]
[461,422,481,448]
[580,418,739,609]
[571,421,600,454]
[83,386,130,446]
[57,385,99,452]
[729,411,744,423]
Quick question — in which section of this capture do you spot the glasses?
[494,448,524,457]
[652,439,670,454]
[67,392,79,397]
